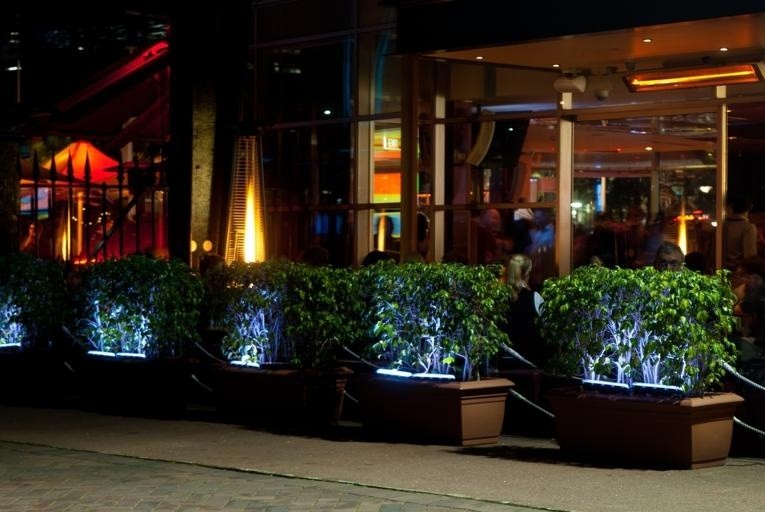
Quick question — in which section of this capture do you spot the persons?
[492,253,558,375]
[200,255,228,294]
[716,268,765,460]
[360,178,765,279]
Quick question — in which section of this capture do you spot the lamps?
[623,65,763,95]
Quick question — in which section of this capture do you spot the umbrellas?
[9,136,130,260]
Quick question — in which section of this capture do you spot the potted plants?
[344,262,515,446]
[73,262,191,421]
[208,261,347,429]
[540,266,744,469]
[5,258,65,409]
